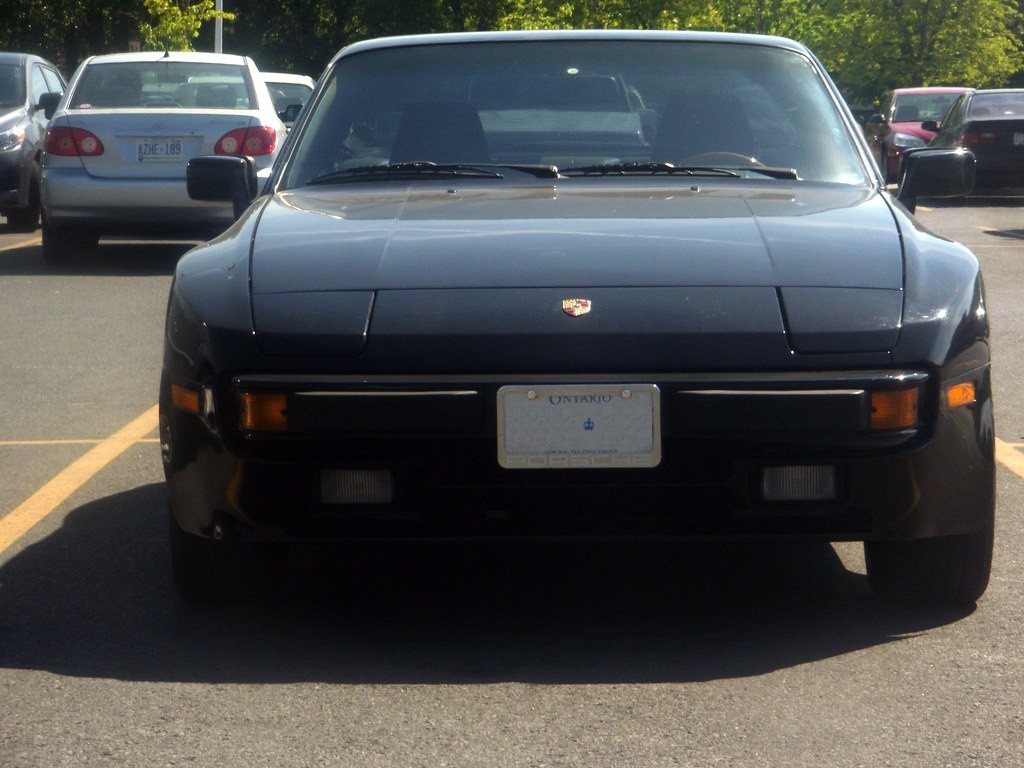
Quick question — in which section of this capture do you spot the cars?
[868,87,977,185]
[37,51,306,257]
[183,73,317,135]
[0,52,69,234]
[925,88,1024,201]
[158,29,994,613]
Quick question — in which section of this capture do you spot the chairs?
[893,105,918,121]
[641,86,756,169]
[105,69,142,104]
[193,83,239,107]
[383,100,496,167]
